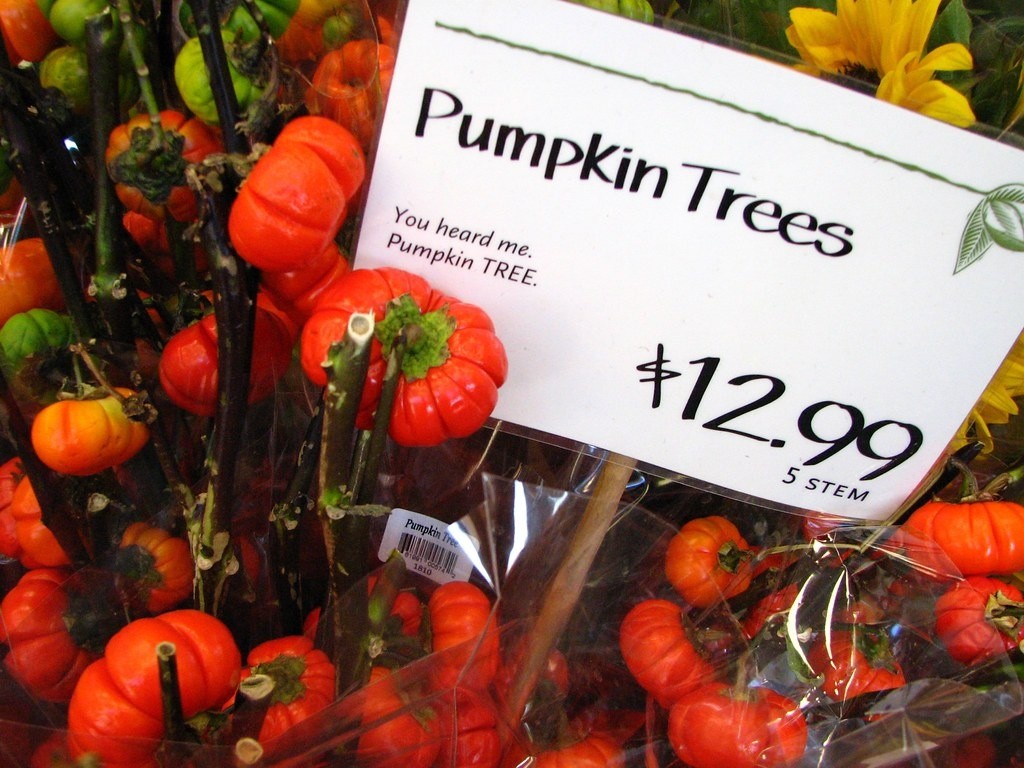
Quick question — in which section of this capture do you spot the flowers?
[683,0,1024,469]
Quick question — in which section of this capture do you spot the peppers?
[0,0,1024,768]
